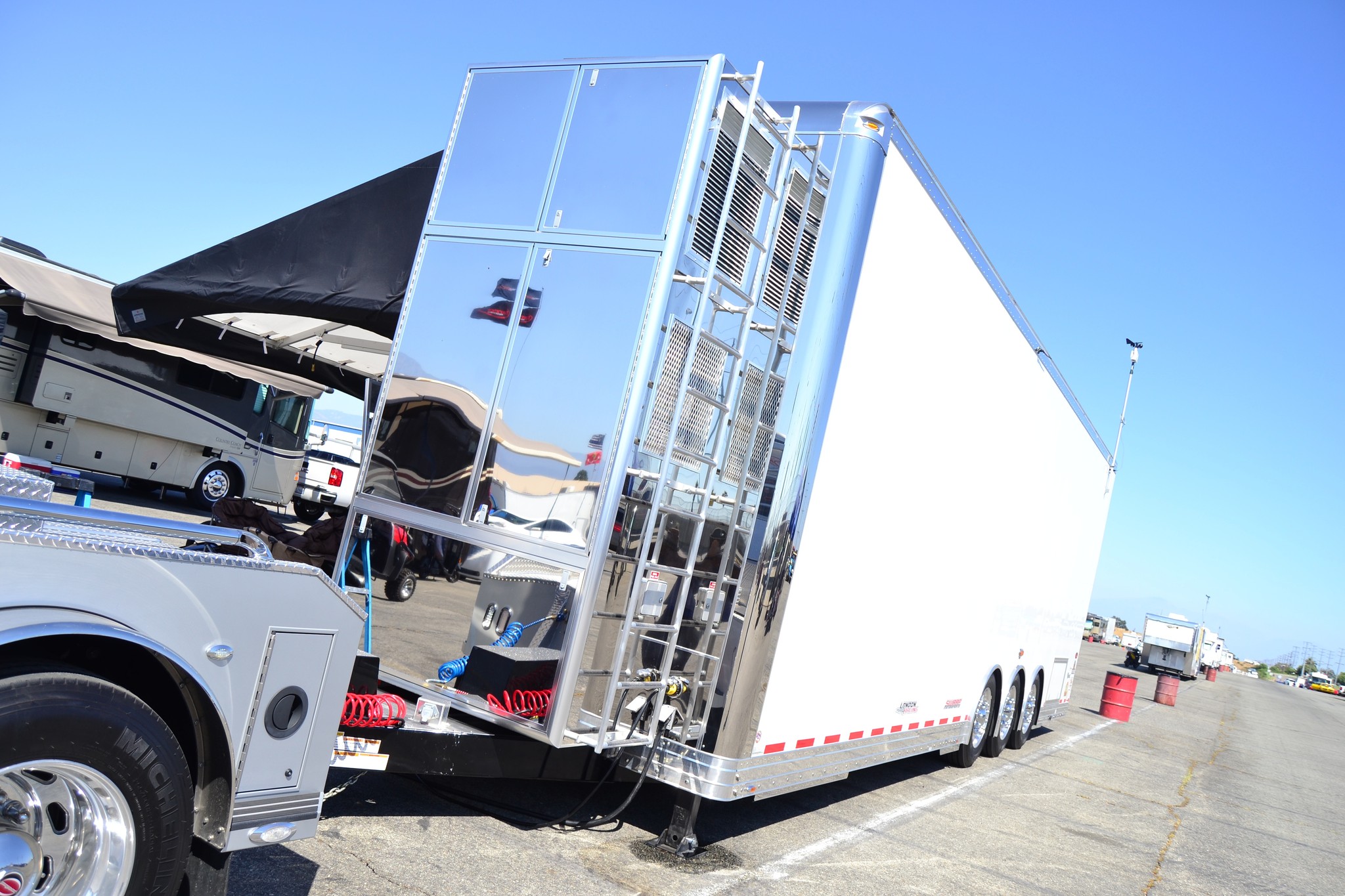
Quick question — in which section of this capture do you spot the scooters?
[1124,647,1141,669]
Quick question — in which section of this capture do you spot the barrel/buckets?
[1203,666,1209,674]
[1088,636,1094,643]
[1224,666,1231,672]
[1153,673,1180,706]
[1098,671,1138,723]
[1218,665,1225,672]
[1206,668,1217,681]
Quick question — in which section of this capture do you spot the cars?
[1311,682,1335,694]
[1284,678,1296,686]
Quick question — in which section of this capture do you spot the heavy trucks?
[0,49,1144,896]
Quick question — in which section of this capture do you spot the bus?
[0,248,332,511]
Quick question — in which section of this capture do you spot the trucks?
[1082,615,1116,645]
